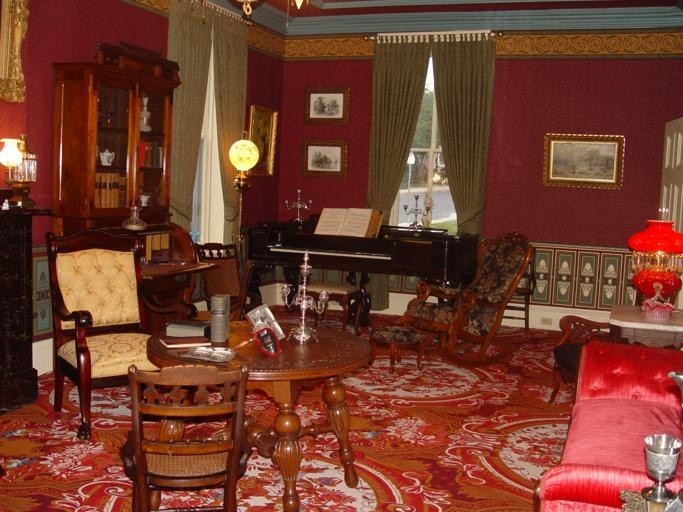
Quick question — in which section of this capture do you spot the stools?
[307,282,363,336]
[369,325,433,374]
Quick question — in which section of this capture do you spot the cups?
[138,195,152,206]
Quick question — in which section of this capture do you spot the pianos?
[241,213,477,328]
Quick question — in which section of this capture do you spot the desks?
[139,260,217,321]
[609,304,683,349]
[147,321,376,512]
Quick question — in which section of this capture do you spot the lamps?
[229,130,260,261]
[0,138,23,180]
[627,220,683,322]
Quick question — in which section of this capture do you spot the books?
[158,336,212,348]
[313,208,374,238]
[166,320,211,337]
[210,294,230,342]
[95,174,119,208]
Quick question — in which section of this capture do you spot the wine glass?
[642,432,681,504]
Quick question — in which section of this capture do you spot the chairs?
[400,231,535,363]
[45,231,161,438]
[543,315,621,404]
[503,250,538,334]
[121,362,252,512]
[196,242,262,314]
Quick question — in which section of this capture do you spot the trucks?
[435,146,448,176]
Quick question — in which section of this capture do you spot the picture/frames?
[542,133,625,191]
[303,137,348,177]
[248,106,279,177]
[303,87,351,124]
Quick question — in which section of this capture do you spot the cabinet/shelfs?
[51,42,181,219]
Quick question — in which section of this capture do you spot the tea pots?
[98,149,115,166]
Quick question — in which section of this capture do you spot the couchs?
[537,339,683,512]
